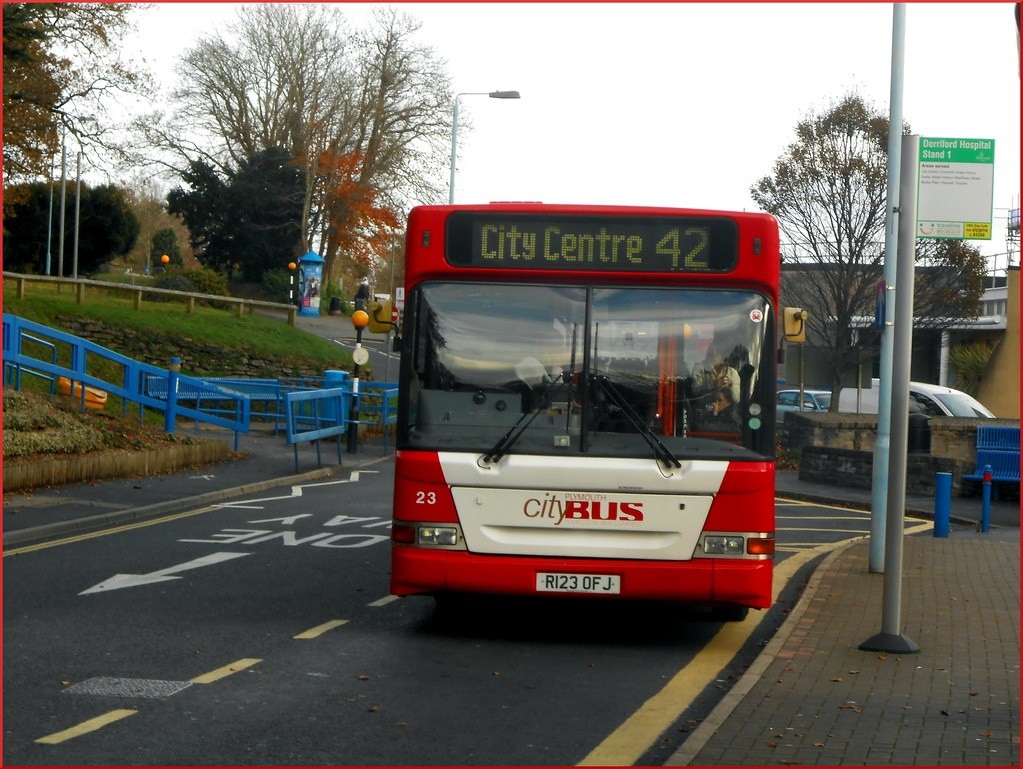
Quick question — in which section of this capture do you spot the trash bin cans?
[321,370,352,441]
[329,297,342,314]
[908,414,932,454]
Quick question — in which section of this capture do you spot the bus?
[368,201,808,622]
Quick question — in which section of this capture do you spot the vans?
[838,379,996,418]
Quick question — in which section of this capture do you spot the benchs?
[963,426,1020,502]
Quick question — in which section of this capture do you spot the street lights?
[449,90,521,204]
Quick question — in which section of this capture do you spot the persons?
[692,339,745,436]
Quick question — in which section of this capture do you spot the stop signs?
[392,308,400,323]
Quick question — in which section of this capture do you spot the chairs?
[593,354,689,376]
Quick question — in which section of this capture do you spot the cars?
[777,390,831,435]
